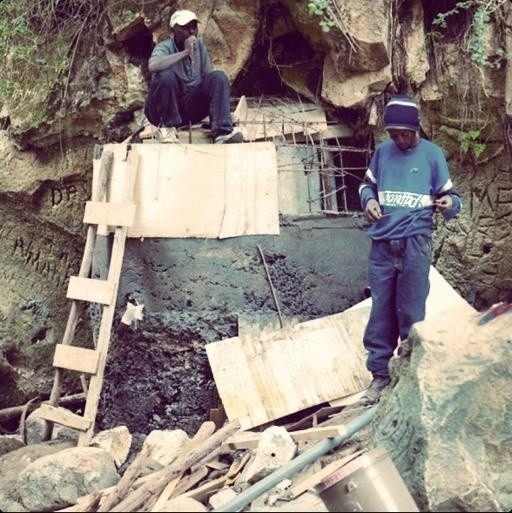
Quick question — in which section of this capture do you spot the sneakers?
[213,129,243,144]
[159,127,180,143]
[362,377,389,406]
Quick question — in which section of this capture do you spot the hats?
[381,94,420,131]
[169,8,201,29]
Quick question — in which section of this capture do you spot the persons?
[357,95,463,406]
[143,9,243,145]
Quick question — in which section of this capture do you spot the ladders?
[39,148,141,448]
